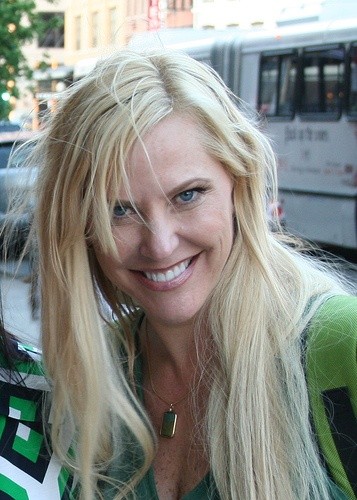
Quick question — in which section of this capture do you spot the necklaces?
[144,315,215,439]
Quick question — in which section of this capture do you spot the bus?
[73,19,357,253]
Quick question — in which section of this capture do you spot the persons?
[3,50,356,500]
